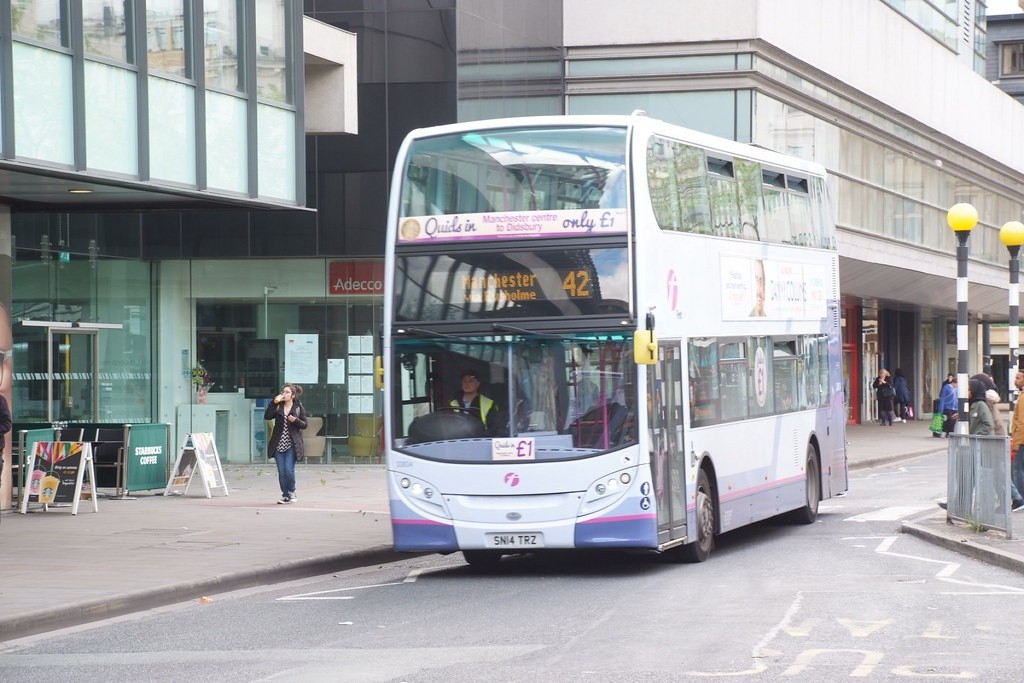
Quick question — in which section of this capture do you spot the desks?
[324,436,349,464]
[92,441,124,447]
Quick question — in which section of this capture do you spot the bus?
[376,108,851,565]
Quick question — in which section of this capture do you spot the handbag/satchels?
[929,412,944,435]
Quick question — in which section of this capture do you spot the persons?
[0,395,12,486]
[939,373,1024,511]
[939,373,957,437]
[445,370,504,437]
[750,259,767,318]
[264,384,309,504]
[873,369,911,426]
[1013,370,1024,499]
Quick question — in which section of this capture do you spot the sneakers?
[277,491,298,505]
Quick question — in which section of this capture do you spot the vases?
[198,385,207,404]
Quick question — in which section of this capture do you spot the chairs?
[53,429,125,499]
[300,416,326,464]
[348,417,379,463]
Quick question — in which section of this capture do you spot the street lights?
[944,200,1024,517]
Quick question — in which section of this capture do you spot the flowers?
[192,366,211,386]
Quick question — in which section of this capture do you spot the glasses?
[281,390,292,394]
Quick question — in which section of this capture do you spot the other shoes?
[1011,498,1023,513]
[939,502,948,508]
[891,415,907,424]
